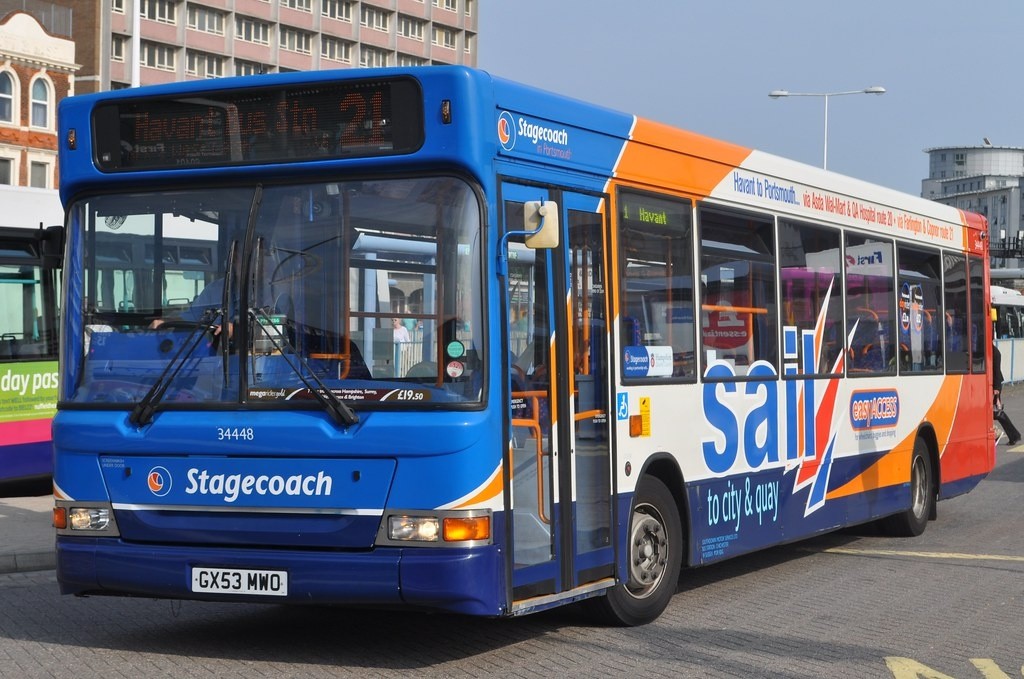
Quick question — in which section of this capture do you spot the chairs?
[642,282,983,378]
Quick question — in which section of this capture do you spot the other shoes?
[995,432,1004,446]
[1007,436,1021,445]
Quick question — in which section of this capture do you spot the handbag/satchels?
[994,391,1005,420]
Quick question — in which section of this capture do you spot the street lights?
[768,86,886,171]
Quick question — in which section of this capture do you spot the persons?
[401,310,421,331]
[146,228,291,349]
[391,317,411,351]
[992,346,1022,445]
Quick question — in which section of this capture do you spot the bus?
[990,286,1024,340]
[57,67,997,626]
[0,186,601,477]
